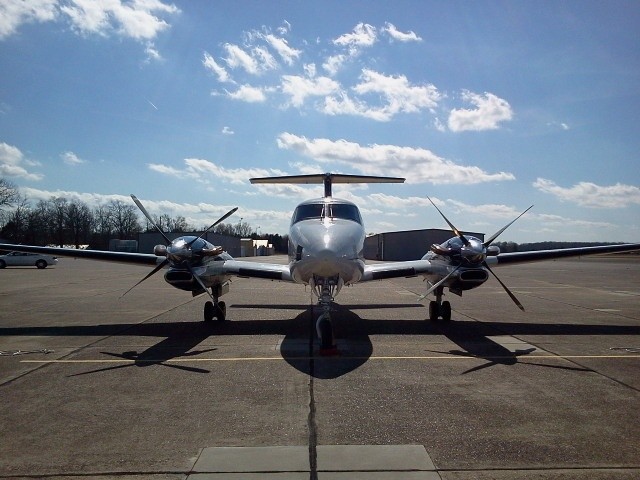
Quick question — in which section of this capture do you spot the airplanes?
[0,173,640,349]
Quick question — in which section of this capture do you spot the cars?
[0,250,58,269]
